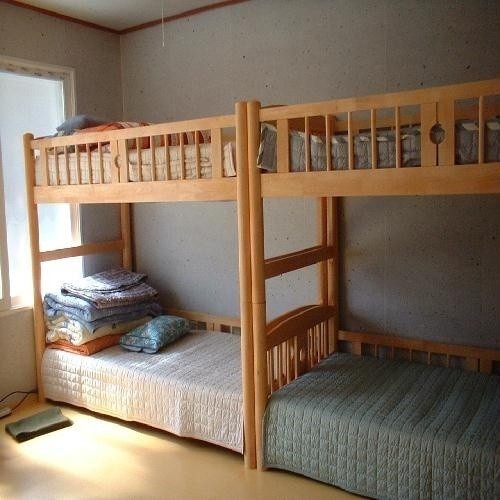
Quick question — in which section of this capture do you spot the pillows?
[118,315,195,353]
[56,113,114,133]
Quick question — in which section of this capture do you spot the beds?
[21,98,256,471]
[251,78,500,499]
